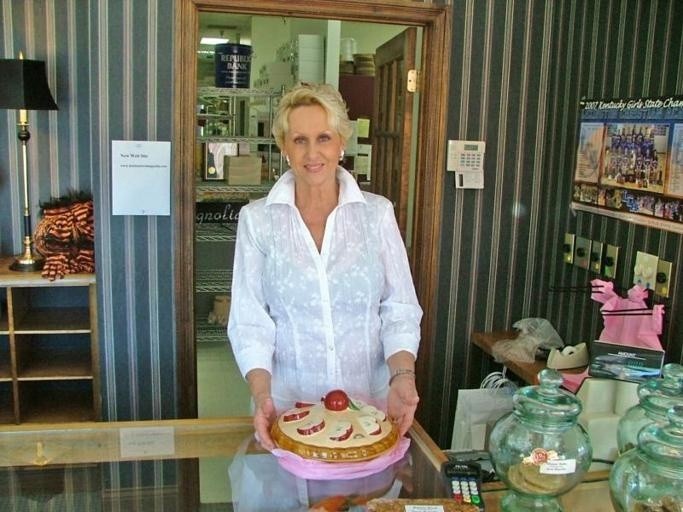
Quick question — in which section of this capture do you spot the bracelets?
[388,366,418,387]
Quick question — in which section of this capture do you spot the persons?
[224,79,424,452]
[636,194,683,222]
[225,431,417,512]
[606,125,662,189]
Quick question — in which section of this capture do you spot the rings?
[391,417,399,421]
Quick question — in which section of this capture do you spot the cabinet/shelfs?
[194,85,287,343]
[0,257,103,425]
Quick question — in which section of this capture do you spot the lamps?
[1,51,59,272]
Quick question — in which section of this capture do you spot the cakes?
[271,390,399,462]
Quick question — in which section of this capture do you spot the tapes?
[562,347,577,356]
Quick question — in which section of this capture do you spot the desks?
[1,407,456,510]
[473,330,546,389]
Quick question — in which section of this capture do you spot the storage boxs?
[224,154,262,190]
